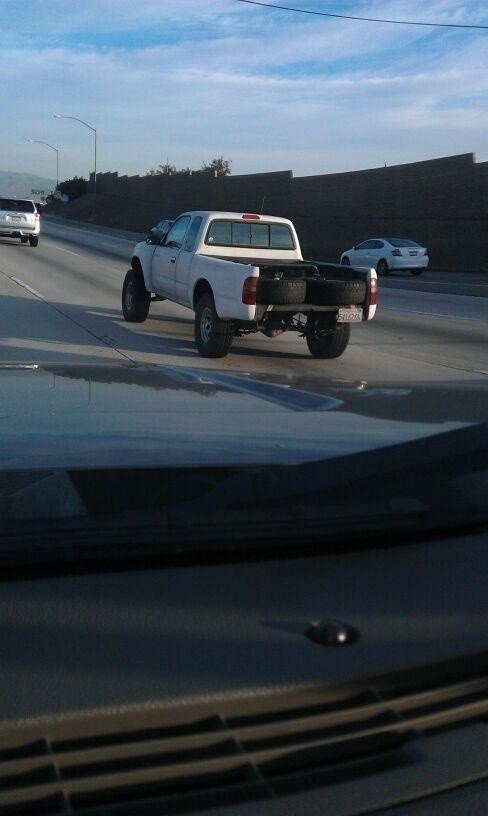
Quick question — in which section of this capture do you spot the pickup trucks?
[119,205,383,362]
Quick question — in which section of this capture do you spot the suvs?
[0,194,40,246]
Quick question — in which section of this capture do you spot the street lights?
[26,111,99,189]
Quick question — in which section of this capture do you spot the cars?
[340,235,430,278]
